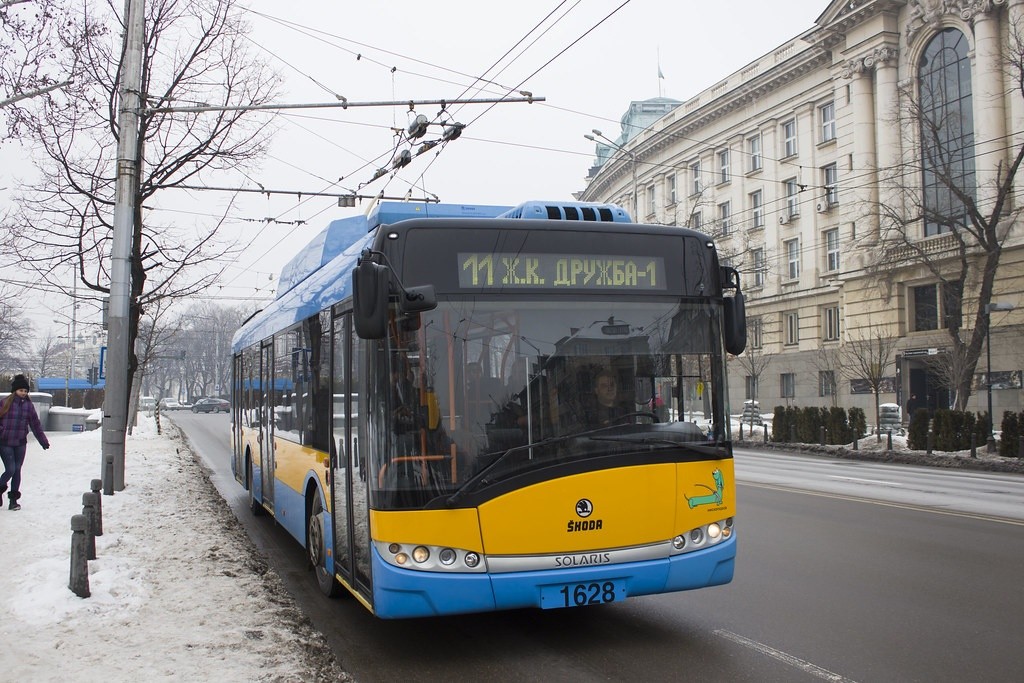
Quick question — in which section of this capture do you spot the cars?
[180,402,194,410]
[192,398,231,414]
[139,397,155,411]
[158,398,181,411]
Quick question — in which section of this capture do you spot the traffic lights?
[87,369,91,383]
[94,369,99,384]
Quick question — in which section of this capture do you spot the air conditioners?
[778,213,791,225]
[816,201,829,213]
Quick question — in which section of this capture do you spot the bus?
[228,196,747,621]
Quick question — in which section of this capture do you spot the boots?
[0,481,8,506]
[7,491,21,510]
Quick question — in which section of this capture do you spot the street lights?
[54,318,66,377]
[986,302,1014,452]
[583,127,638,223]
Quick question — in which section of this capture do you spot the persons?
[0,375,50,510]
[582,370,632,432]
[907,394,918,423]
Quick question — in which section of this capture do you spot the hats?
[11,375,30,393]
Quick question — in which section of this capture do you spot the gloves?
[43,446,49,450]
